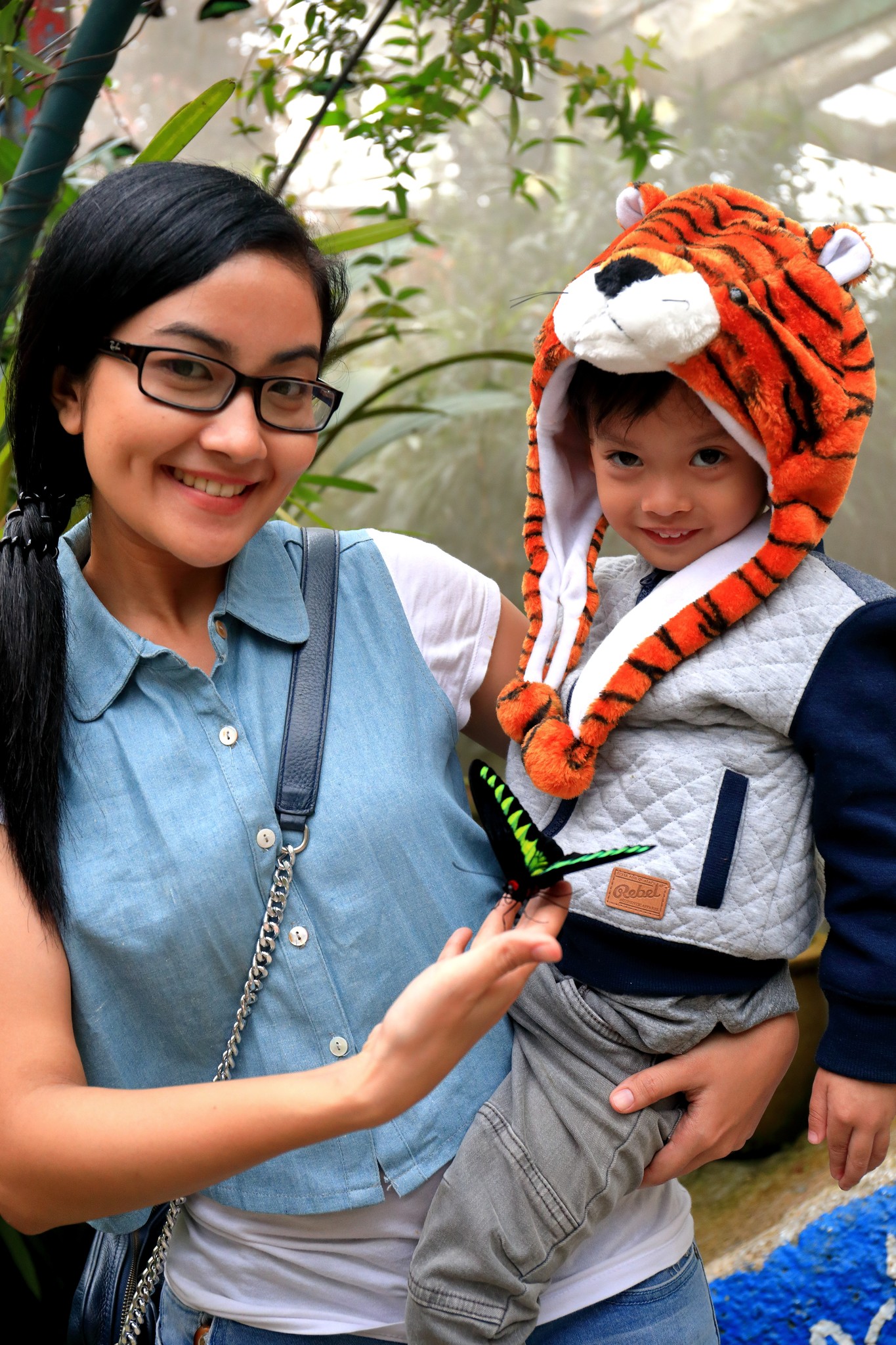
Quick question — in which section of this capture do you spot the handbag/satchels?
[64,1204,165,1345]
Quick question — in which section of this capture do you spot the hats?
[495,183,875,801]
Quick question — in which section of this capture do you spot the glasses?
[101,338,343,434]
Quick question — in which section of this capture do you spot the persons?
[405,178,896,1345]
[0,160,896,1345]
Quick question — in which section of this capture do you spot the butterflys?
[451,758,660,931]
